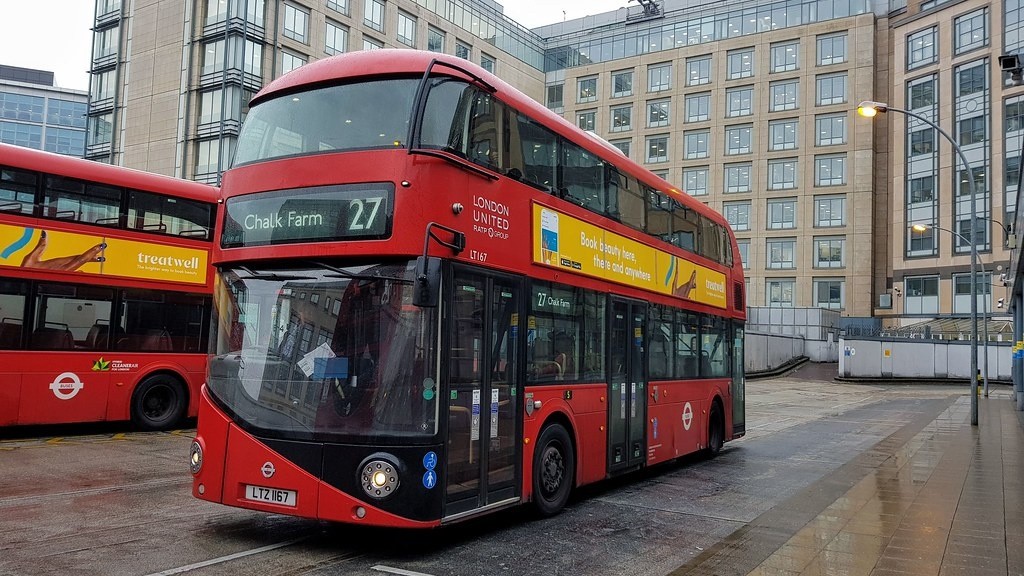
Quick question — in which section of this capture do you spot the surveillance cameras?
[999,274,1006,281]
[998,53,1024,80]
[998,298,1004,303]
[997,303,1003,308]
[894,287,897,290]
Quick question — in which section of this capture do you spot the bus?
[190,46,744,529]
[0,140,222,436]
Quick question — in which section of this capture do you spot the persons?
[21,230,107,273]
[541,332,574,380]
[671,258,696,299]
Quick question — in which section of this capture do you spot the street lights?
[859,99,978,427]
[912,223,990,399]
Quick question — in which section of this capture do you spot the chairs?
[95,218,119,226]
[524,340,712,380]
[0,202,23,213]
[139,224,167,233]
[53,211,75,220]
[0,317,165,352]
[179,230,207,239]
